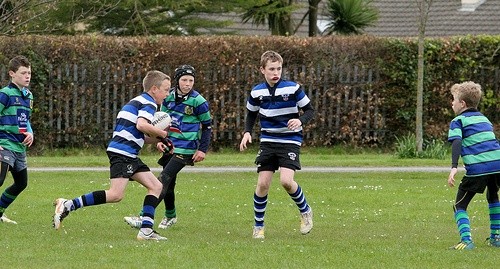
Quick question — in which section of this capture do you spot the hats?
[174,64,195,81]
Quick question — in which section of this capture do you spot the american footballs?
[143,111,172,138]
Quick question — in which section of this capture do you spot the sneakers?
[252,225,265,238]
[137,230,167,241]
[158,217,177,229]
[124,216,144,228]
[300,205,313,235]
[0,216,16,225]
[54,198,66,230]
[449,242,473,251]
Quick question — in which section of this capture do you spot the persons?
[52,71,174,241]
[0,55,34,223]
[240,51,313,239]
[124,65,212,228]
[448,82,500,250]
